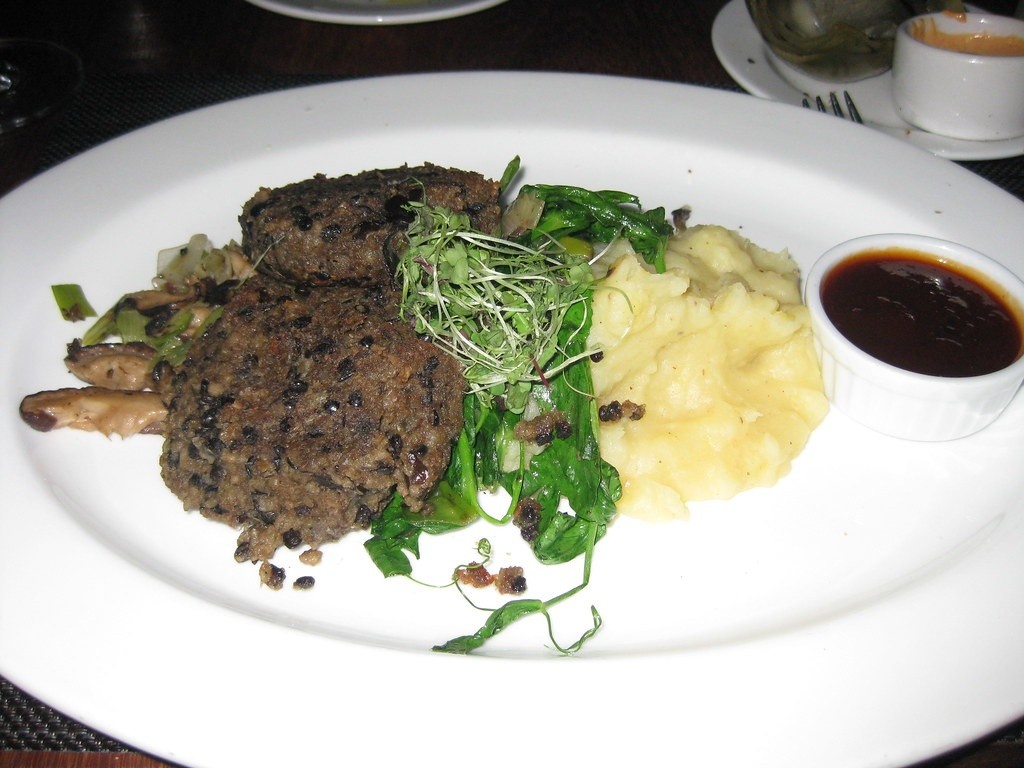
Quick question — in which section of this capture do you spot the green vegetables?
[362,163,690,653]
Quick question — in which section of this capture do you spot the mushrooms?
[19,341,172,438]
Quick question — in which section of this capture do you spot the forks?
[802,90,863,125]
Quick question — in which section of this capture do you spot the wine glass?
[0,37,88,135]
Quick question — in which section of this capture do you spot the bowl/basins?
[892,12,1024,140]
[804,233,1024,442]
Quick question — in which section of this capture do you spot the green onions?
[50,231,245,368]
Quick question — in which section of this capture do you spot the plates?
[711,0,1024,161]
[0,71,1024,768]
[249,0,507,26]
